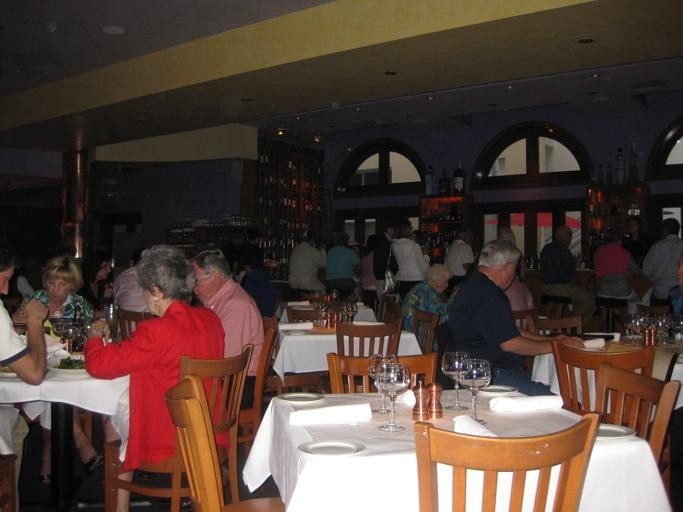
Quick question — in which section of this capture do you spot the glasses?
[190,274,209,284]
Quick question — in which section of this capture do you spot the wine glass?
[441,352,470,412]
[460,360,491,426]
[367,353,398,416]
[624,312,672,347]
[378,365,410,432]
[314,301,358,328]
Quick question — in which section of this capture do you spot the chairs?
[287,306,320,324]
[513,309,537,329]
[413,413,603,512]
[104,343,255,512]
[551,340,654,427]
[119,308,151,340]
[527,314,583,335]
[413,308,440,354]
[327,351,439,394]
[164,373,286,512]
[262,314,321,402]
[594,363,682,463]
[336,316,404,357]
[238,327,279,494]
[378,294,397,325]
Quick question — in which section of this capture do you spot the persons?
[241,257,274,317]
[0,246,48,386]
[6,272,35,315]
[113,247,153,338]
[391,219,430,299]
[594,227,640,308]
[445,226,474,295]
[360,235,377,305]
[72,196,108,259]
[498,227,526,280]
[505,278,534,330]
[324,227,360,296]
[400,263,460,354]
[189,249,264,410]
[622,215,651,268]
[287,230,326,294]
[83,244,232,512]
[77,240,113,319]
[540,227,591,288]
[13,255,103,485]
[667,254,683,339]
[447,239,585,397]
[374,225,392,299]
[642,219,683,306]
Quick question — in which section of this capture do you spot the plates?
[277,391,324,403]
[48,354,92,377]
[472,383,519,394]
[0,364,19,378]
[595,422,638,441]
[297,437,367,456]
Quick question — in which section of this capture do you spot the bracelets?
[95,328,105,335]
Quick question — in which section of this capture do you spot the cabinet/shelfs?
[418,193,474,249]
[581,181,650,261]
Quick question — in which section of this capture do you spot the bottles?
[439,168,451,195]
[423,228,458,249]
[428,383,443,418]
[452,158,467,194]
[424,164,439,197]
[644,329,651,346]
[412,380,429,420]
[73,306,82,321]
[650,329,657,347]
[418,203,462,224]
[226,214,254,225]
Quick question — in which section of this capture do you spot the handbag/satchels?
[384,248,399,293]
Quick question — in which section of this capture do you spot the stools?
[595,297,627,331]
[541,294,571,317]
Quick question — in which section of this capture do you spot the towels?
[395,389,415,406]
[352,320,383,325]
[452,414,496,442]
[489,394,564,415]
[287,301,309,306]
[583,339,606,349]
[279,322,313,330]
[288,402,373,428]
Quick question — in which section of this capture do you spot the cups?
[104,303,119,341]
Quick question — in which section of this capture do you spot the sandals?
[39,472,53,484]
[77,454,106,473]
